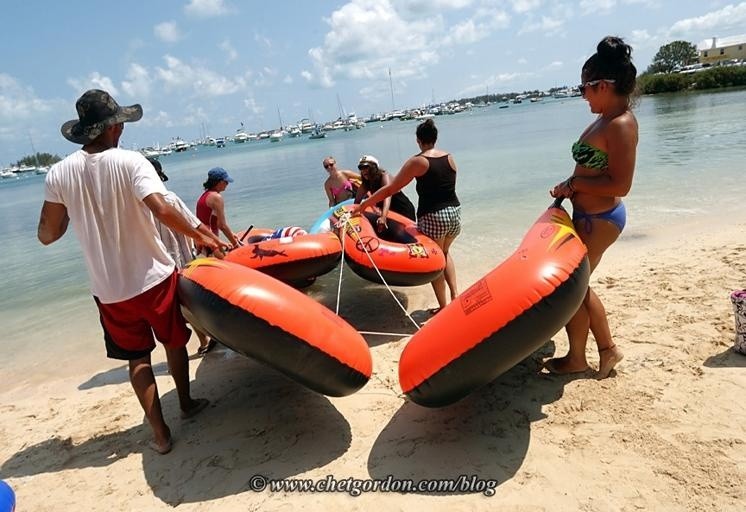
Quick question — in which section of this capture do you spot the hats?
[208,167,233,182]
[358,155,379,169]
[151,159,168,181]
[61,89,142,145]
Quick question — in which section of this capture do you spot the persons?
[37,90,207,457]
[349,120,462,315]
[194,167,246,257]
[354,155,417,234]
[141,157,233,356]
[323,156,362,207]
[546,34,638,378]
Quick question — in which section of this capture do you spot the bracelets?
[567,175,578,193]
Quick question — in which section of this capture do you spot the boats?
[2,67,585,179]
[329,204,445,287]
[309,197,367,235]
[172,255,373,398]
[397,193,603,408]
[209,226,343,288]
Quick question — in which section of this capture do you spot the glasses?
[578,79,616,95]
[325,163,333,168]
